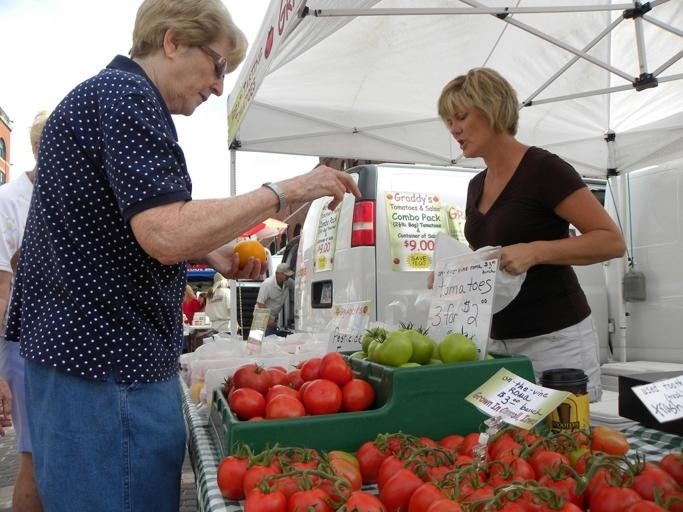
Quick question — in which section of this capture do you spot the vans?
[295,162,609,384]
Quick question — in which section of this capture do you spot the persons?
[5,0,360,511]
[254,262,294,336]
[181,285,199,352]
[0,111,56,511]
[205,272,240,337]
[197,293,206,312]
[428,67,625,403]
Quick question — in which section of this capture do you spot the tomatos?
[217,427,683,512]
[221,321,495,421]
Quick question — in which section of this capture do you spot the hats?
[275,262,295,277]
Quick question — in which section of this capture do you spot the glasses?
[191,37,230,81]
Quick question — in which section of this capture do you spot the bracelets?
[261,180,287,213]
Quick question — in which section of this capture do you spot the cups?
[541,367,590,430]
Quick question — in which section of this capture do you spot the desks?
[188,397,681,512]
[173,355,224,434]
